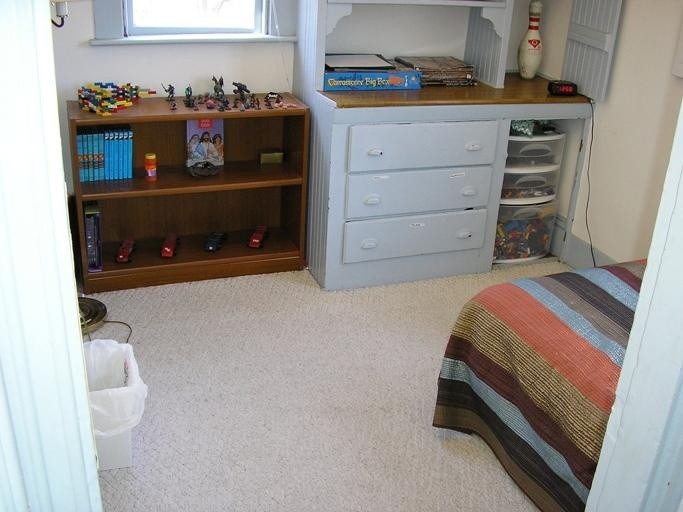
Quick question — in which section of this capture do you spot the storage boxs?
[492,120,567,268]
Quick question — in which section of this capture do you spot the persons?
[187,132,224,168]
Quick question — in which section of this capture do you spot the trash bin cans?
[84,339,139,471]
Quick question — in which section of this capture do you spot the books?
[324,53,396,72]
[185,118,226,168]
[395,54,476,88]
[76,124,132,183]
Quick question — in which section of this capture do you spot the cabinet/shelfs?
[64,91,311,296]
[293,119,499,291]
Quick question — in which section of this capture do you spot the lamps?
[49,0,74,29]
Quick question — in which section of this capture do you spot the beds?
[433,258,648,510]
